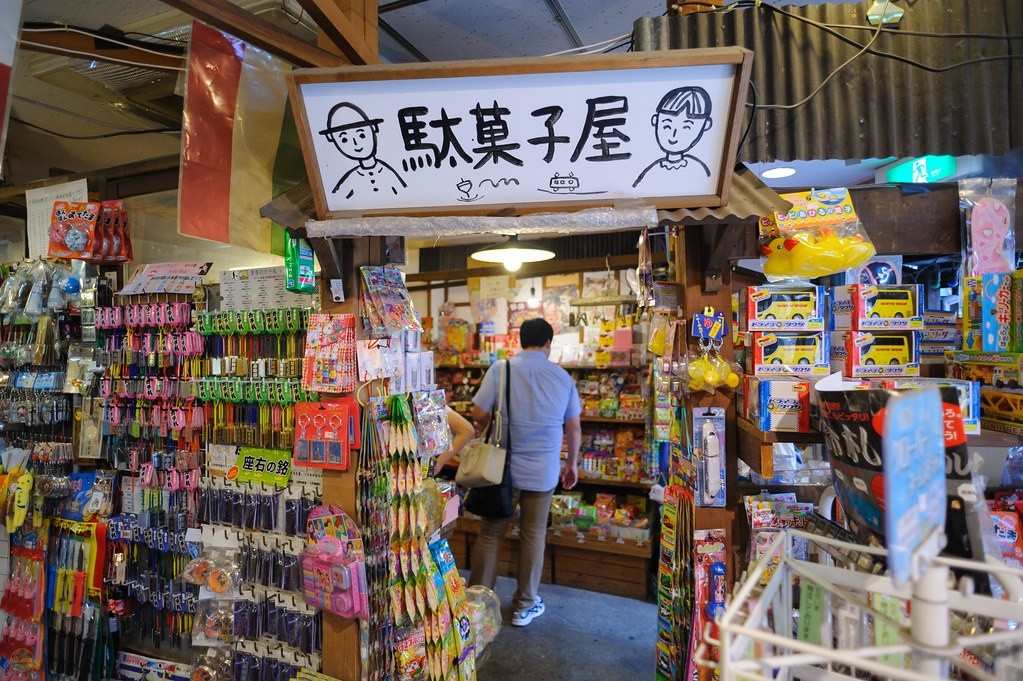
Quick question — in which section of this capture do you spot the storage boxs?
[735,255,1023,437]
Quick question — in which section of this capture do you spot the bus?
[865,289,913,319]
[763,335,819,364]
[757,292,815,321]
[861,335,909,365]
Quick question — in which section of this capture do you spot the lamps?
[470,238,555,272]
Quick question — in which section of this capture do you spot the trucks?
[981,390,1023,422]
[963,364,1023,389]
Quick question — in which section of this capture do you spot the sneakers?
[512,594,545,626]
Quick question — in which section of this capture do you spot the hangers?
[568,255,654,329]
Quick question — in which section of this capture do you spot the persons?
[434,404,475,477]
[469,317,582,627]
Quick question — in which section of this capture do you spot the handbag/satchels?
[463,453,513,518]
[455,444,506,487]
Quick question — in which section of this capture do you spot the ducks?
[761,230,878,278]
